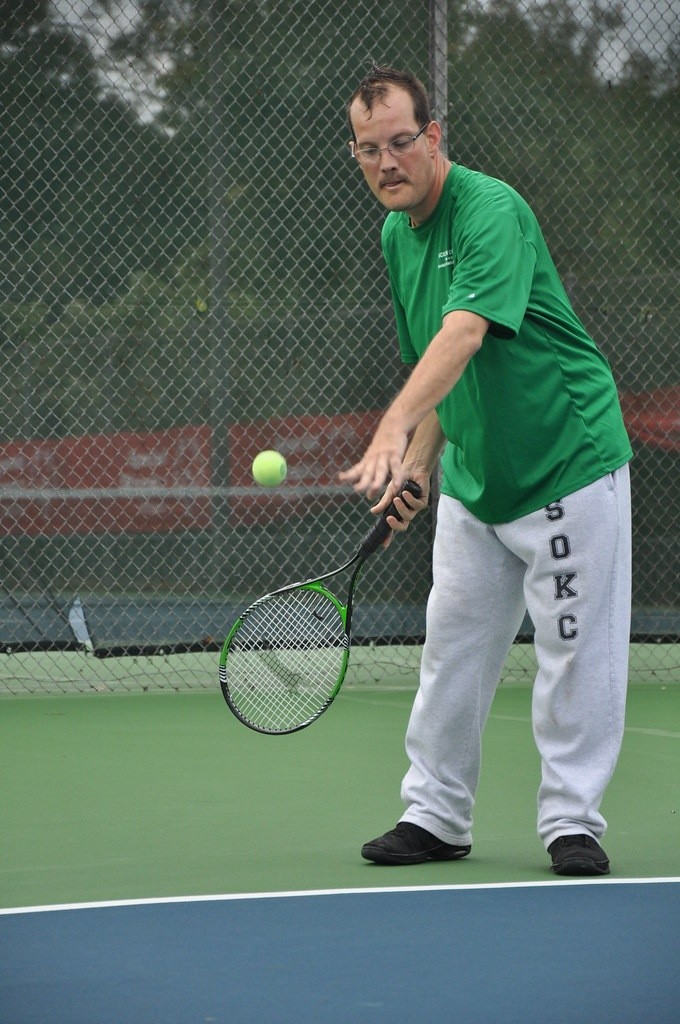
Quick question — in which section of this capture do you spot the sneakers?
[360,822,472,865]
[547,834,610,876]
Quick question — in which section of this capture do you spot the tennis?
[251,450,288,488]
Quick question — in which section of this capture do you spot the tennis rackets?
[218,479,423,735]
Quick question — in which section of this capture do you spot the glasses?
[349,121,430,166]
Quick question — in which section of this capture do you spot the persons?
[337,72,634,875]
